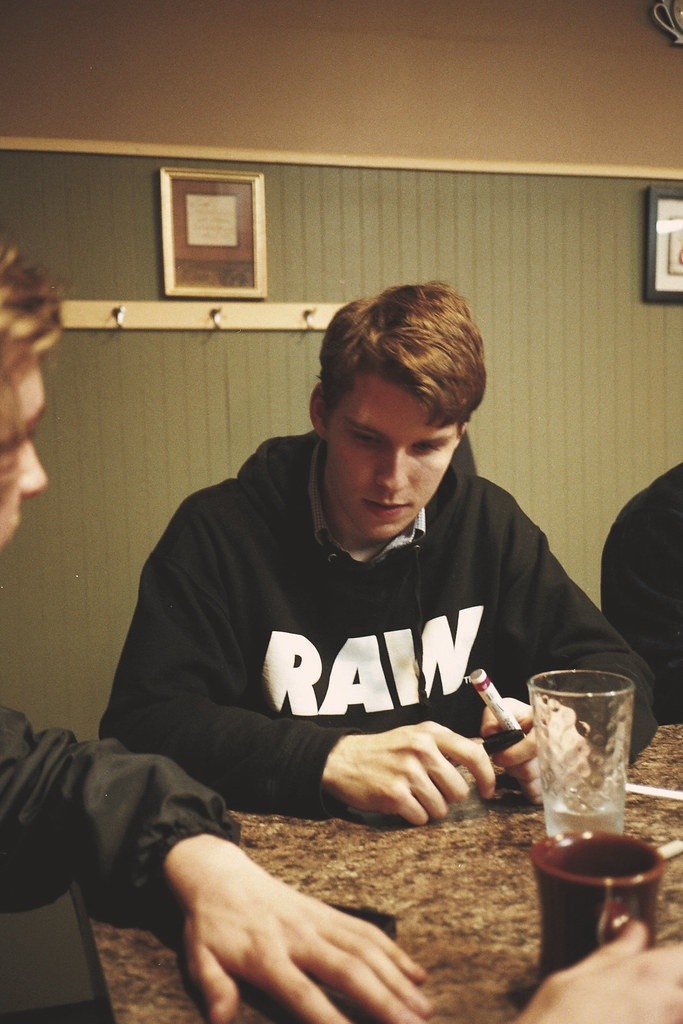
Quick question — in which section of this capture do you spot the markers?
[433,729,524,767]
[470,668,527,741]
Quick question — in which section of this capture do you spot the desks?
[70,723,683,1022]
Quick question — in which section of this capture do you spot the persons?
[97,280,656,830]
[0,251,683,1024]
[600,463,682,724]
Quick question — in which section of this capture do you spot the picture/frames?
[159,165,268,300]
[644,185,683,304]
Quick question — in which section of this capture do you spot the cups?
[526,667,638,845]
[528,831,665,983]
